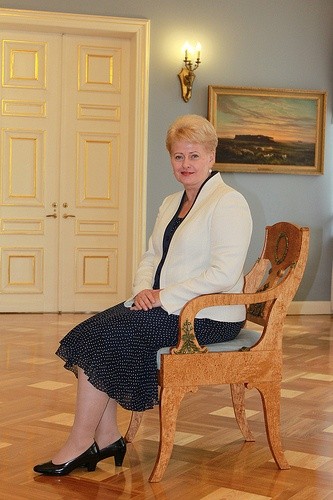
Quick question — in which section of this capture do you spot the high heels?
[96,436,126,467]
[34,441,100,475]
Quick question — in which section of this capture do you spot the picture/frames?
[206,84,328,177]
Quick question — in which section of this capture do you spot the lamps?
[176,42,202,102]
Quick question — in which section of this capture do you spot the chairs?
[122,220,310,483]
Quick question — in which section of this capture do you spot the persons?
[33,115,253,477]
